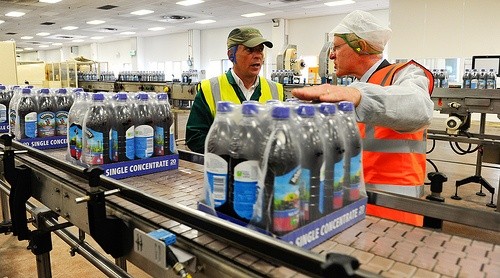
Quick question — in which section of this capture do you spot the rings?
[326,89,330,94]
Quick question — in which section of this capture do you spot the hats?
[329,9,392,51]
[227,28,273,48]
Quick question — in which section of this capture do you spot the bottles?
[462,69,496,89]
[201,99,360,235]
[332,70,357,85]
[78,71,166,83]
[0,85,170,167]
[180,70,206,84]
[271,68,300,84]
[431,68,448,87]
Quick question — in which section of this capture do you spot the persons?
[185,26,286,154]
[291,10,435,227]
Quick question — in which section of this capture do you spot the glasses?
[327,39,358,52]
